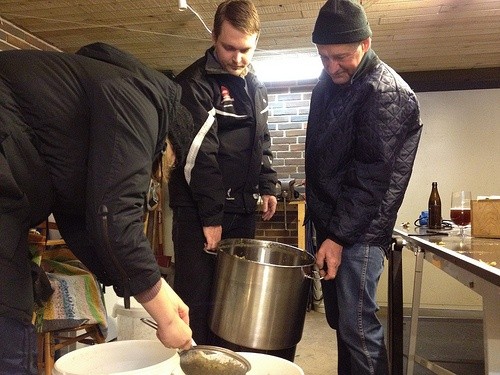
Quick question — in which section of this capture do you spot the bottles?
[428,182,441,229]
[419,211,428,228]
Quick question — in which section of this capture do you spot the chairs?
[35,212,107,375]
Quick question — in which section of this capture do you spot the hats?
[312,0,372,44]
[167,104,194,157]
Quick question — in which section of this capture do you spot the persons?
[302,0,423,375]
[168,0,278,345]
[0,41,194,375]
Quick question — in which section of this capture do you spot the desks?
[391,221,500,375]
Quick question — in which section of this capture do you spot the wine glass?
[450,189,473,238]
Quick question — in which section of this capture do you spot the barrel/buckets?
[52,295,304,375]
[203,238,326,350]
